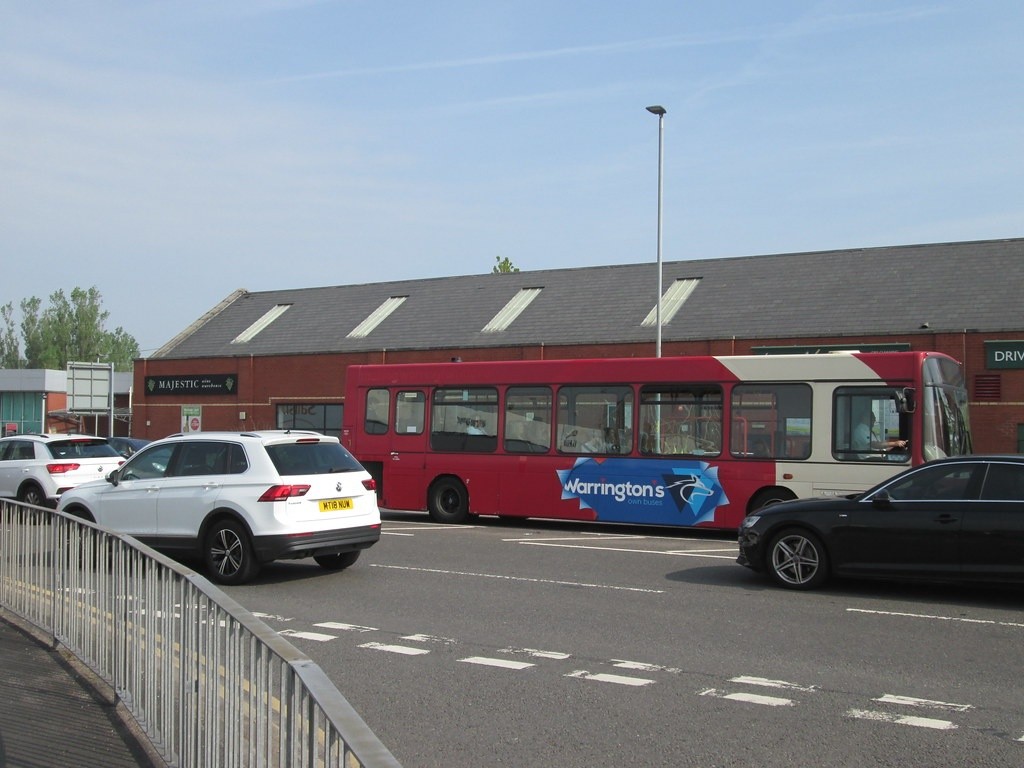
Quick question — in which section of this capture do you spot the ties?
[870,429,879,442]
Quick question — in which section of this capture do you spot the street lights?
[644,104,668,456]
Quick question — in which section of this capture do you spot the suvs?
[0,431,134,526]
[55,427,382,587]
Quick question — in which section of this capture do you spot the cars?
[80,436,173,474]
[736,454,1024,593]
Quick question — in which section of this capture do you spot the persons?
[182,447,212,474]
[467,414,488,435]
[601,428,618,454]
[933,474,969,492]
[511,420,524,439]
[851,409,906,460]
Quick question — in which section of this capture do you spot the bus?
[341,343,975,532]
